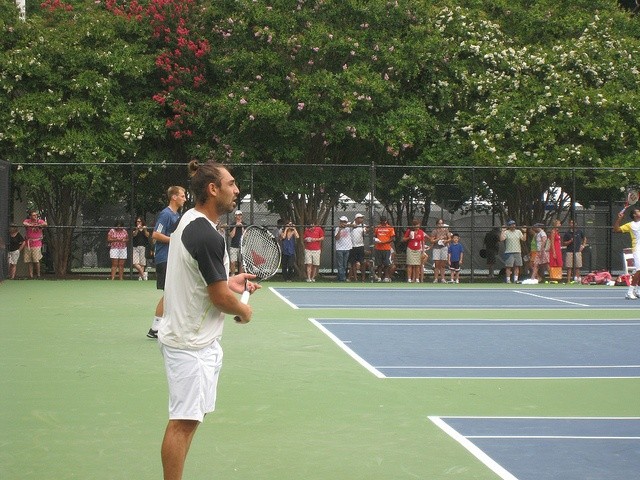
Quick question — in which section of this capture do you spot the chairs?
[622,252,639,277]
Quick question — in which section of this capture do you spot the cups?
[410,231,414,240]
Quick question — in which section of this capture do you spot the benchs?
[344,252,408,279]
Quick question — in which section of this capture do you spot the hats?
[508,220,516,226]
[355,213,364,219]
[235,210,242,215]
[340,216,348,222]
[8,224,18,232]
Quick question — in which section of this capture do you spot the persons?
[347,213,370,281]
[530,223,550,284]
[303,222,325,283]
[448,233,464,284]
[158,161,263,480]
[23,211,47,280]
[548,220,563,284]
[146,186,186,338]
[107,220,129,280]
[370,247,396,282]
[229,210,246,275]
[430,219,451,283]
[373,216,395,282]
[500,220,527,284]
[6,224,25,279]
[613,209,640,300]
[279,221,300,281]
[561,219,586,285]
[132,216,149,281]
[401,221,424,283]
[335,216,352,282]
[216,218,226,240]
[529,226,542,283]
[484,227,500,279]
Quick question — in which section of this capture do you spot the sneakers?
[138,274,142,280]
[143,272,148,280]
[625,293,637,300]
[634,291,640,297]
[146,328,158,338]
